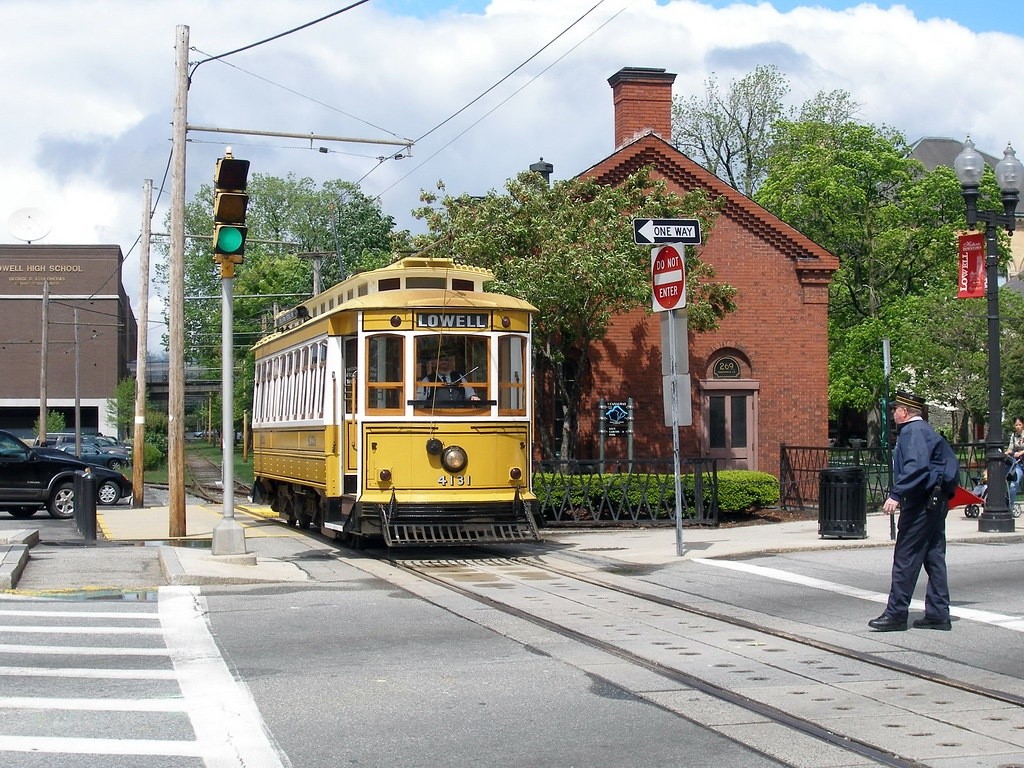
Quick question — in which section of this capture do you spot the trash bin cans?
[817,465,868,538]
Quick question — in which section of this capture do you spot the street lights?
[954,131,1024,531]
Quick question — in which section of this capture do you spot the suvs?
[0,429,94,519]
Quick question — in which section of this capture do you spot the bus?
[247,202,549,549]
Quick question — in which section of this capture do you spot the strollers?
[963,450,1024,519]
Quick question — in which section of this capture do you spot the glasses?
[892,405,908,412]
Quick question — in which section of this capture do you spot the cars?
[0,432,133,506]
[193,428,218,437]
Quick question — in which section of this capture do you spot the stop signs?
[648,241,688,314]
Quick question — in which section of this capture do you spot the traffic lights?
[209,154,253,278]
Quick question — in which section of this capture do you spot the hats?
[889,390,926,411]
[433,350,447,359]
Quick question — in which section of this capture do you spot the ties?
[442,376,450,393]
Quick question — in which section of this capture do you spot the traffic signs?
[632,218,702,247]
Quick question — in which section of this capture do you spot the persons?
[1005,416,1024,509]
[416,352,480,409]
[972,468,988,498]
[866,391,959,630]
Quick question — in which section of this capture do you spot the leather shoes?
[913,616,951,631]
[869,613,907,631]
